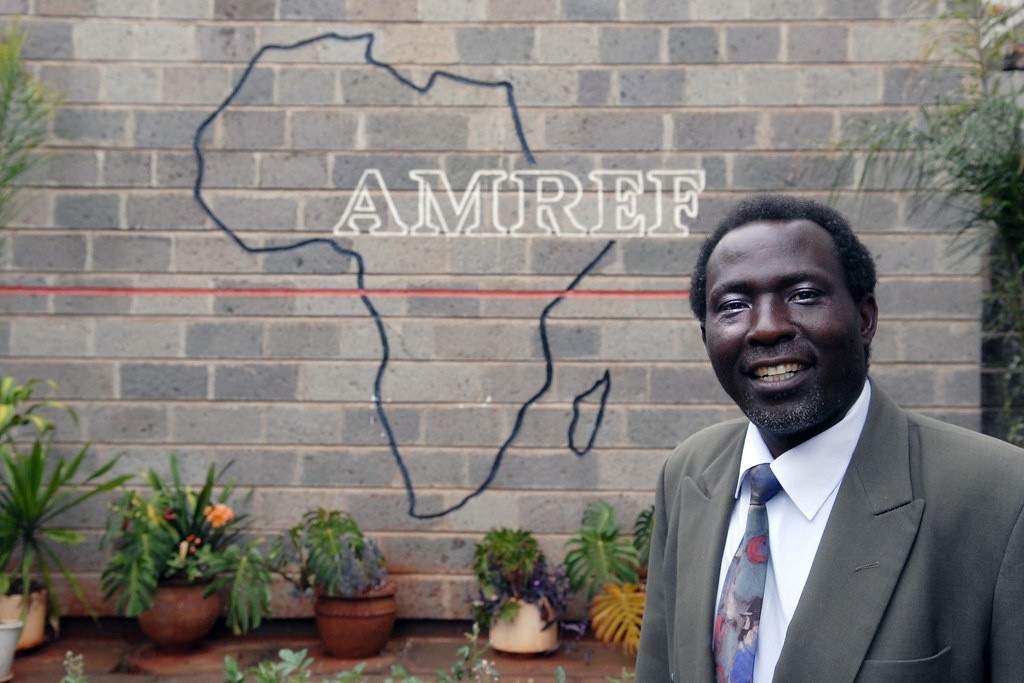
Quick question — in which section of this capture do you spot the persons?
[635,193,1024,683]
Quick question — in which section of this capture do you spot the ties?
[712,464,781,683]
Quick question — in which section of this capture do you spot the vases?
[136,573,223,646]
[316,583,398,657]
[0,620,25,683]
[490,603,557,653]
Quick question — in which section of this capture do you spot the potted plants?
[564,500,655,658]
[0,376,136,651]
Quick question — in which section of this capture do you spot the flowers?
[96,452,264,617]
[467,526,590,665]
[271,508,390,598]
